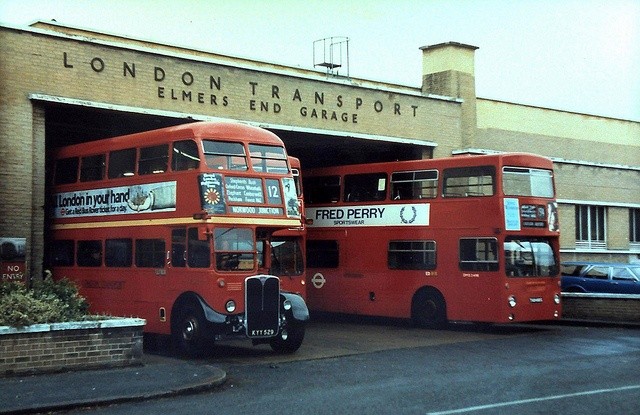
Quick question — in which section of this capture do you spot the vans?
[559,261,640,294]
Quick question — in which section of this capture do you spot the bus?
[45,122,310,359]
[297,153,561,328]
[207,152,306,231]
